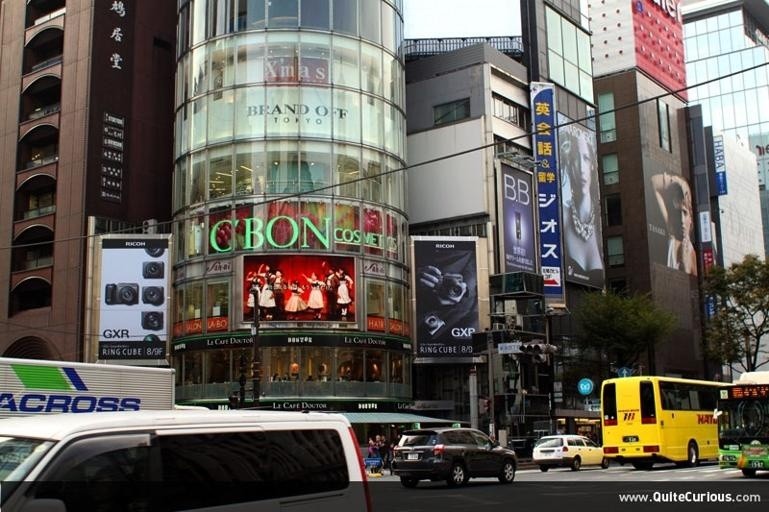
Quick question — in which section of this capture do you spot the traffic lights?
[519,343,547,365]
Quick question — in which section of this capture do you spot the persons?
[190,178,202,203]
[559,135,605,287]
[209,183,250,198]
[415,248,479,341]
[366,432,401,476]
[650,172,697,277]
[178,347,403,383]
[244,263,353,320]
[557,423,602,445]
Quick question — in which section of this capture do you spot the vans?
[1,408,374,512]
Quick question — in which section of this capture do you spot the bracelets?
[663,171,674,191]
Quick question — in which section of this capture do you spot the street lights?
[249,287,260,407]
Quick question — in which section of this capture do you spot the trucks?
[0,355,178,446]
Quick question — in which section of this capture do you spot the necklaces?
[568,195,594,241]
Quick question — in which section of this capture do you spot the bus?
[716,370,769,478]
[599,374,737,471]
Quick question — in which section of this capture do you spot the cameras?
[105,282,139,305]
[143,261,164,279]
[435,272,463,298]
[141,311,164,331]
[141,285,164,306]
[422,312,444,336]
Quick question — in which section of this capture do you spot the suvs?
[390,427,518,490]
[531,434,610,472]
